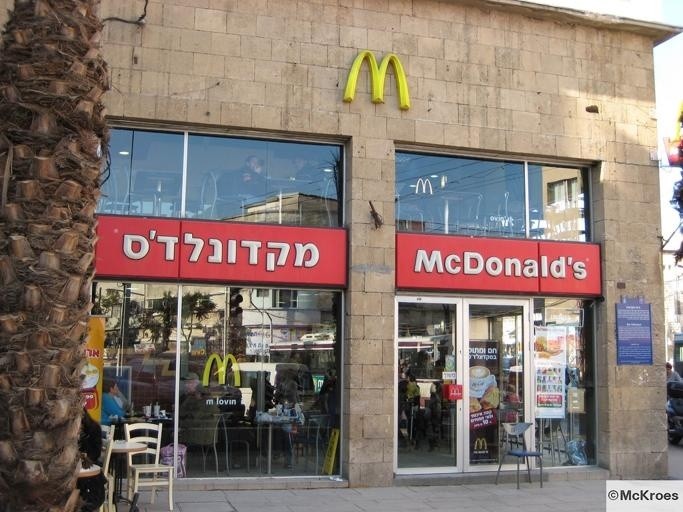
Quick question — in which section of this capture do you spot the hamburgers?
[547,340,563,355]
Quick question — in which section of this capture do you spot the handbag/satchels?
[158,443,186,478]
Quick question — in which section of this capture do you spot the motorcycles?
[667,381,683,446]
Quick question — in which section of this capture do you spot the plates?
[469,374,497,399]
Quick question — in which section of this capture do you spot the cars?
[116,352,220,411]
[502,353,582,393]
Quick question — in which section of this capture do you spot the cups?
[468,366,491,391]
[143,405,151,418]
[153,405,160,417]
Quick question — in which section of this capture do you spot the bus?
[265,331,447,412]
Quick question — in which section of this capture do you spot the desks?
[92,439,147,511]
[76,462,100,479]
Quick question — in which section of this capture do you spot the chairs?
[122,419,175,510]
[95,439,115,512]
[497,418,547,489]
[97,402,576,476]
[101,163,542,241]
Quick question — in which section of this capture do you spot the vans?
[225,361,317,414]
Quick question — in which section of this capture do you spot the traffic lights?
[230,287,243,317]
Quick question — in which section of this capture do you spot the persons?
[666,362,681,382]
[100,379,132,426]
[162,351,337,469]
[291,154,326,198]
[77,406,107,512]
[237,154,274,196]
